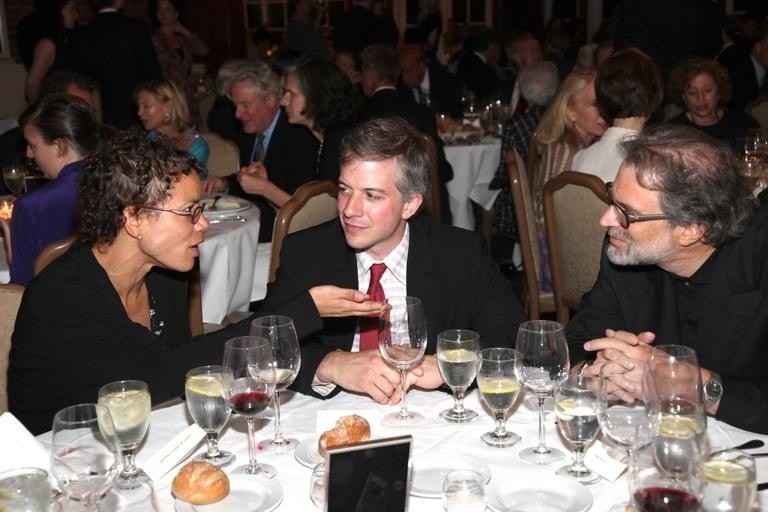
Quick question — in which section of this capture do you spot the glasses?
[606,182,672,229]
[142,202,206,224]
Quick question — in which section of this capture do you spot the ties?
[253,135,265,162]
[360,264,390,352]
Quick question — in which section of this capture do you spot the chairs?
[266,181,341,287]
[200,131,240,177]
[542,170,617,338]
[503,147,556,331]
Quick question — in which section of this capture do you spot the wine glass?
[379,295,428,427]
[552,372,599,483]
[600,377,654,510]
[435,92,512,141]
[245,314,302,454]
[0,164,26,223]
[738,133,768,194]
[95,379,152,488]
[51,403,123,511]
[221,336,279,480]
[435,330,484,424]
[512,319,571,466]
[184,363,237,467]
[630,347,759,509]
[474,348,524,447]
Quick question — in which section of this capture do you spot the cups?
[1,465,51,511]
[440,467,487,511]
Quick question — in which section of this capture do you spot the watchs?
[701,366,725,411]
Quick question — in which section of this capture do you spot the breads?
[318,425,345,459]
[335,413,371,443]
[171,459,231,506]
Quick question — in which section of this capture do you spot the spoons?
[208,195,221,212]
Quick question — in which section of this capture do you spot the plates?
[170,476,284,512]
[294,438,335,465]
[405,454,490,494]
[485,472,593,510]
[199,195,252,214]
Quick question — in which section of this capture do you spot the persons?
[0,0,767,312]
[520,122,768,438]
[2,124,393,436]
[251,111,533,406]
